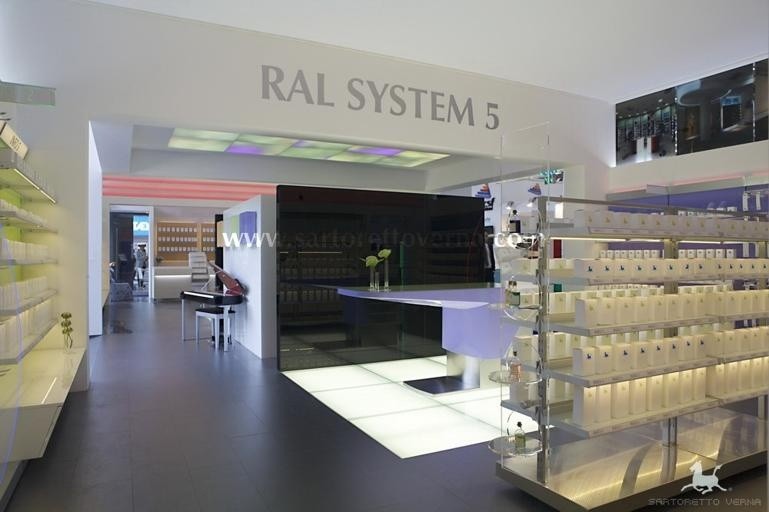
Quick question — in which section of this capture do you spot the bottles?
[508,209,522,235]
[277,229,368,307]
[503,282,514,306]
[514,421,526,451]
[511,281,521,307]
[508,350,521,379]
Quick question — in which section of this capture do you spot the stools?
[195,307,235,349]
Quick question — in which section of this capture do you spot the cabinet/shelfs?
[497,198,769,510]
[1,168,86,461]
[401,230,485,339]
[276,247,370,335]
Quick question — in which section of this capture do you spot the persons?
[136,244,148,289]
[482,231,496,288]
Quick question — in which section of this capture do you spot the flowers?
[378,248,393,260]
[360,255,384,268]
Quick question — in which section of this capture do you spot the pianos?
[179,264,245,349]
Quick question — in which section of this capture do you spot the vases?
[383,258,390,290]
[369,264,376,291]
[374,271,380,292]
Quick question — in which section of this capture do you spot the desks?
[280,280,485,353]
[337,280,539,395]
